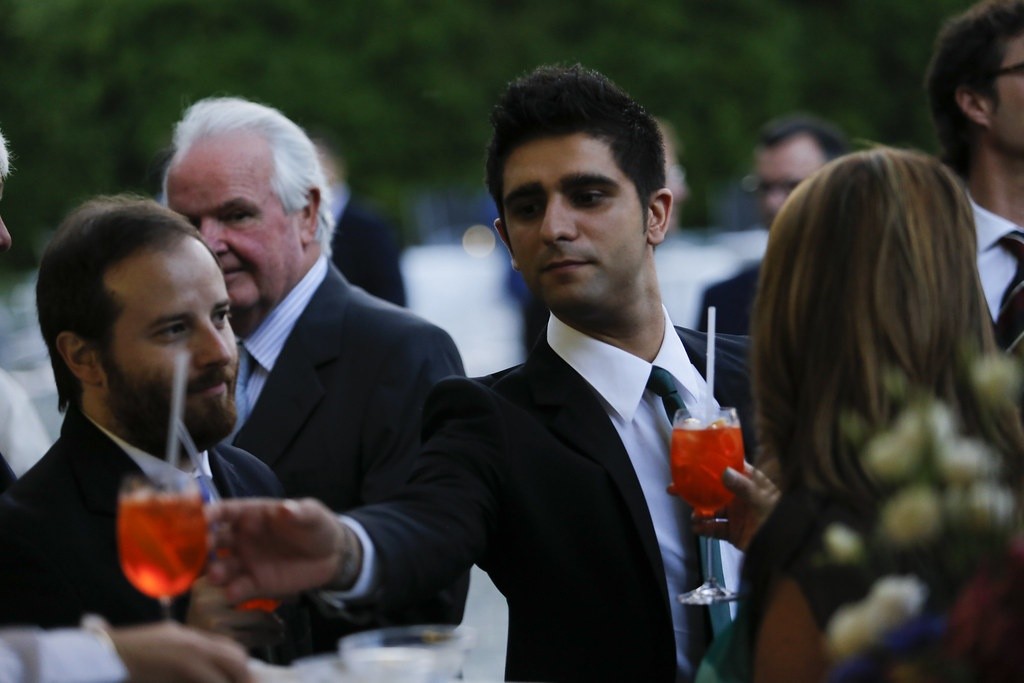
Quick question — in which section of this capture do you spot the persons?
[666,146,1024,682]
[203,62,752,683]
[0,134,255,683]
[700,111,851,338]
[303,123,407,310]
[166,96,470,659]
[923,0,1024,364]
[0,190,318,666]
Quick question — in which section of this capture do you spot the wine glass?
[669,406,747,607]
[114,470,206,624]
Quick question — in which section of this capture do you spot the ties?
[194,477,219,560]
[220,340,257,448]
[645,365,731,641]
[995,232,1024,367]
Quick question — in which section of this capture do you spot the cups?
[338,623,477,683]
